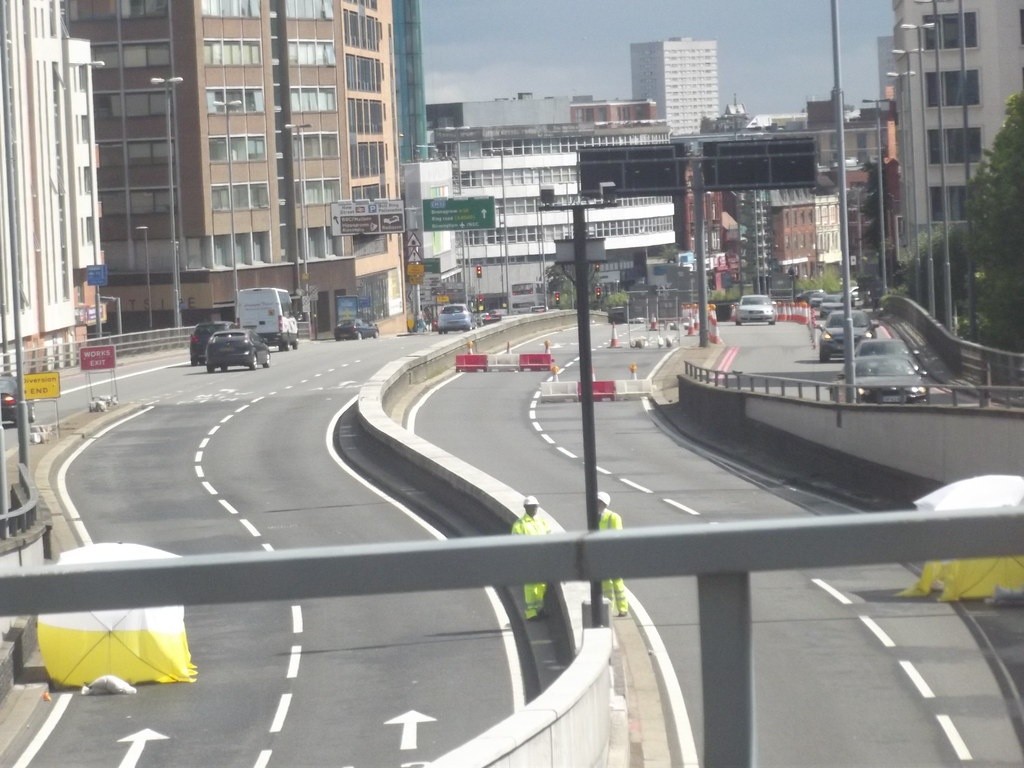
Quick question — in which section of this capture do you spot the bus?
[509,281,553,315]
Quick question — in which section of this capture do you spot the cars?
[438,303,478,335]
[0,375,37,427]
[205,328,272,373]
[332,319,380,341]
[480,309,502,326]
[734,294,777,325]
[794,286,930,405]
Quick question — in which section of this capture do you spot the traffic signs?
[330,198,406,237]
[422,195,497,232]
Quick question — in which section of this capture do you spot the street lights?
[860,22,941,321]
[211,100,243,327]
[491,148,512,315]
[150,76,185,330]
[443,126,471,310]
[284,122,312,341]
[84,60,105,348]
[135,226,153,330]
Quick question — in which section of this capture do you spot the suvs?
[189,320,235,366]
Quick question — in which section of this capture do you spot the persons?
[598,492,628,616]
[512,496,553,620]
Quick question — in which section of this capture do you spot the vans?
[237,287,299,352]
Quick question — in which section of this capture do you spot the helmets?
[523,496,539,506]
[597,491,610,506]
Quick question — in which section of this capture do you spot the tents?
[891,474,1024,600]
[36,541,200,685]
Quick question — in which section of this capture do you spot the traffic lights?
[596,288,601,299]
[477,265,482,278]
[555,293,560,304]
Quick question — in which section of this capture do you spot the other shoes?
[528,616,536,623]
[618,611,627,616]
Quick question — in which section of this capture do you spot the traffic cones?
[544,340,551,354]
[649,317,658,331]
[729,300,809,325]
[551,366,559,382]
[505,341,512,354]
[467,340,473,355]
[606,321,623,349]
[681,303,722,345]
